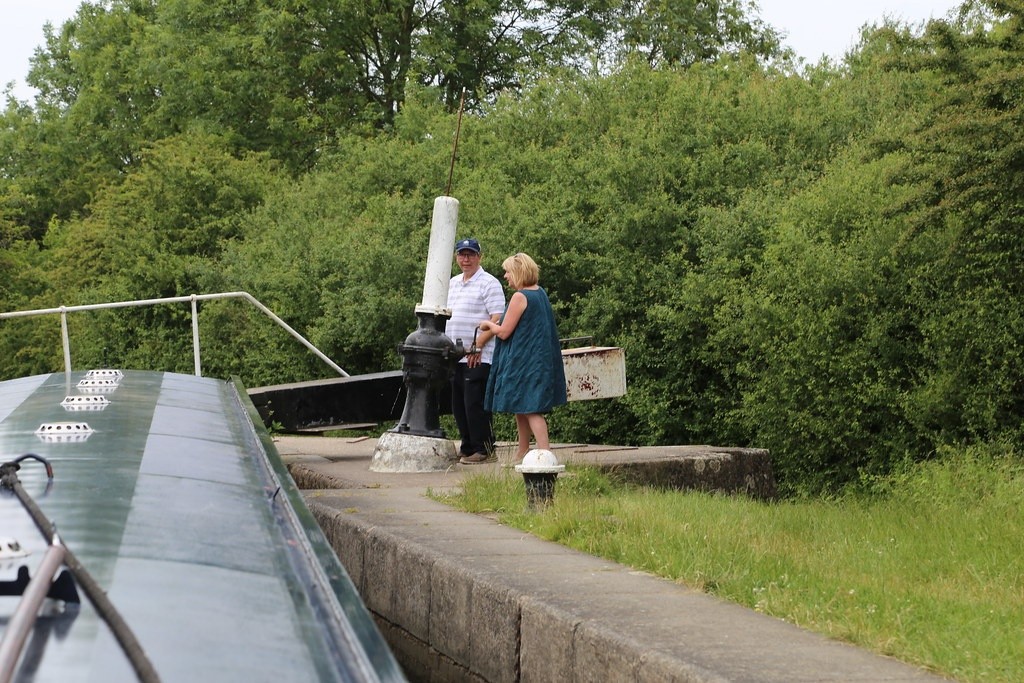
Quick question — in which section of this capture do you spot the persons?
[479,253,567,467]
[445,240,505,464]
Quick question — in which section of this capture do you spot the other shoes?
[460,452,498,464]
[501,458,522,468]
[456,453,467,463]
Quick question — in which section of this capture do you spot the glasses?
[457,252,477,258]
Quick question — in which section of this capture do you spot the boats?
[0,370,408,683]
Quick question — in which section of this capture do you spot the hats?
[456,239,480,253]
